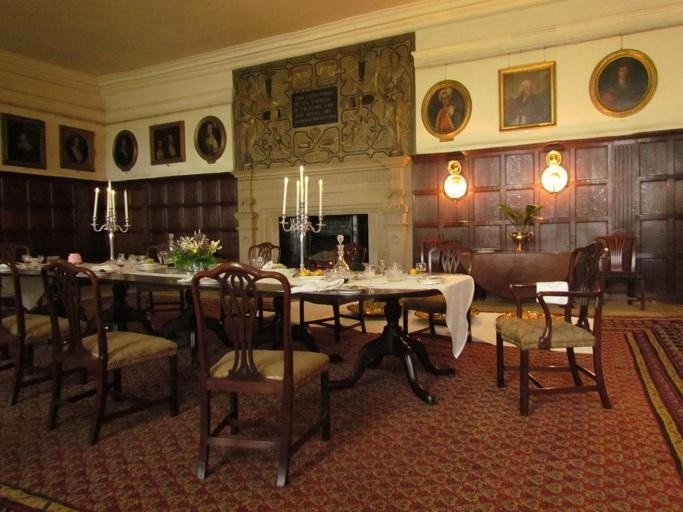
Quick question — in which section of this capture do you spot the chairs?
[497,241,615,418]
[594,234,647,313]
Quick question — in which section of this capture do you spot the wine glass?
[22,253,44,272]
[359,258,439,289]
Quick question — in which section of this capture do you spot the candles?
[281,165,325,219]
[92,177,133,226]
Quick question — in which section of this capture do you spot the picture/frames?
[147,120,188,169]
[192,115,230,167]
[420,78,474,145]
[0,110,49,174]
[112,129,140,173]
[586,46,660,121]
[497,59,560,133]
[58,123,97,174]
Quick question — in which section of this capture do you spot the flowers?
[170,228,225,274]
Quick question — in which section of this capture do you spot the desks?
[462,251,583,301]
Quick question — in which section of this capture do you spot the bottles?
[332,234,350,284]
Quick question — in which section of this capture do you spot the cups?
[113,252,167,276]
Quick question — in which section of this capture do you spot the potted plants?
[498,202,549,256]
